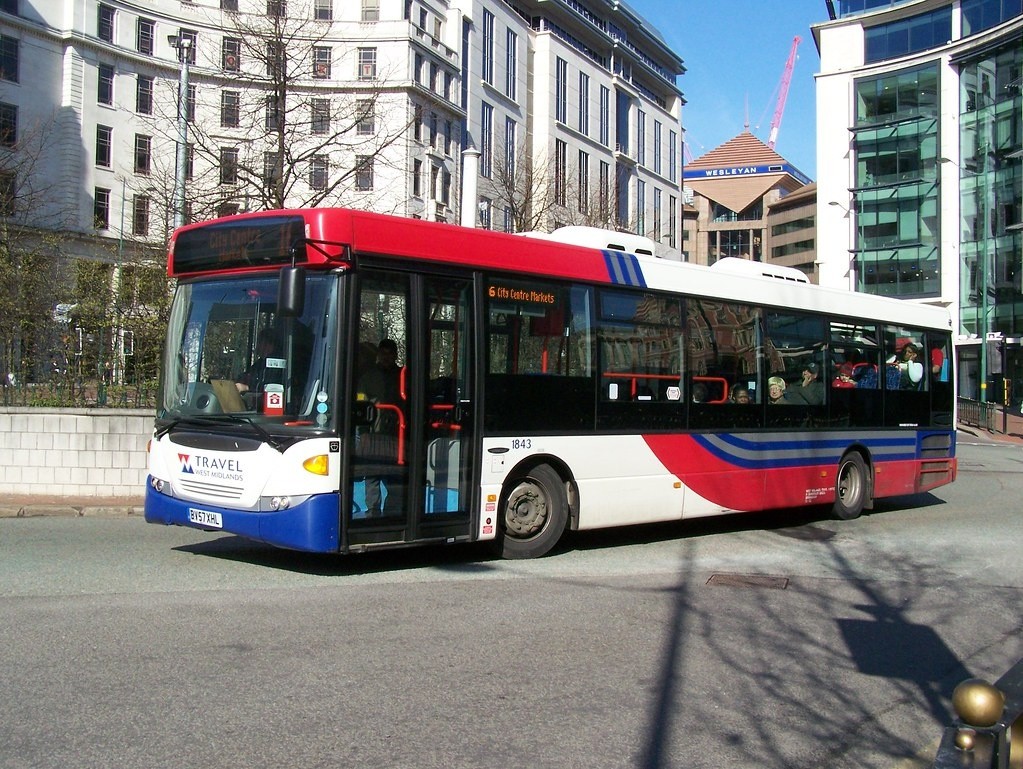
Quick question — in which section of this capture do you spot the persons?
[374,338,404,411]
[608,330,942,405]
[231,329,274,392]
[355,342,391,516]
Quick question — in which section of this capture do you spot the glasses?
[769,388,781,391]
[839,373,849,377]
[735,394,748,398]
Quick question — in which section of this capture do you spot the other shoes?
[365,508,381,519]
[383,508,402,517]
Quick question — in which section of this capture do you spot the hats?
[839,361,853,377]
[800,362,819,374]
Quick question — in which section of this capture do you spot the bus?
[144,209,959,560]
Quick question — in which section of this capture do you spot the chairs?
[935,358,949,382]
[878,363,901,390]
[851,363,878,389]
